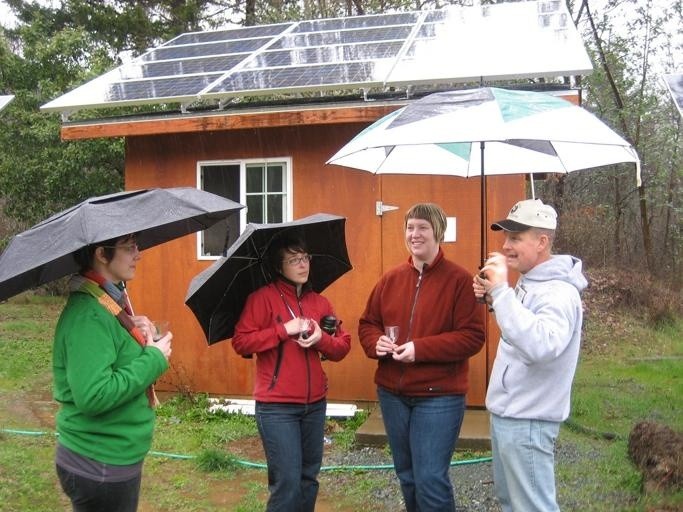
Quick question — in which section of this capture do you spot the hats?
[491,198,558,233]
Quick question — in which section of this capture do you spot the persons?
[472,199,588,512]
[53,234,174,512]
[232,237,351,512]
[357,204,489,512]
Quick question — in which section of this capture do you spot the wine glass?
[384,326,400,354]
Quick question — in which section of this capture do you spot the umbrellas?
[0,185,245,318]
[184,213,353,339]
[324,87,639,305]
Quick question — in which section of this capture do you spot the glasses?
[100,242,139,253]
[281,253,313,266]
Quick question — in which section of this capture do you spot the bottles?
[318,315,343,361]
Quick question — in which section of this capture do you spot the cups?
[149,320,169,343]
